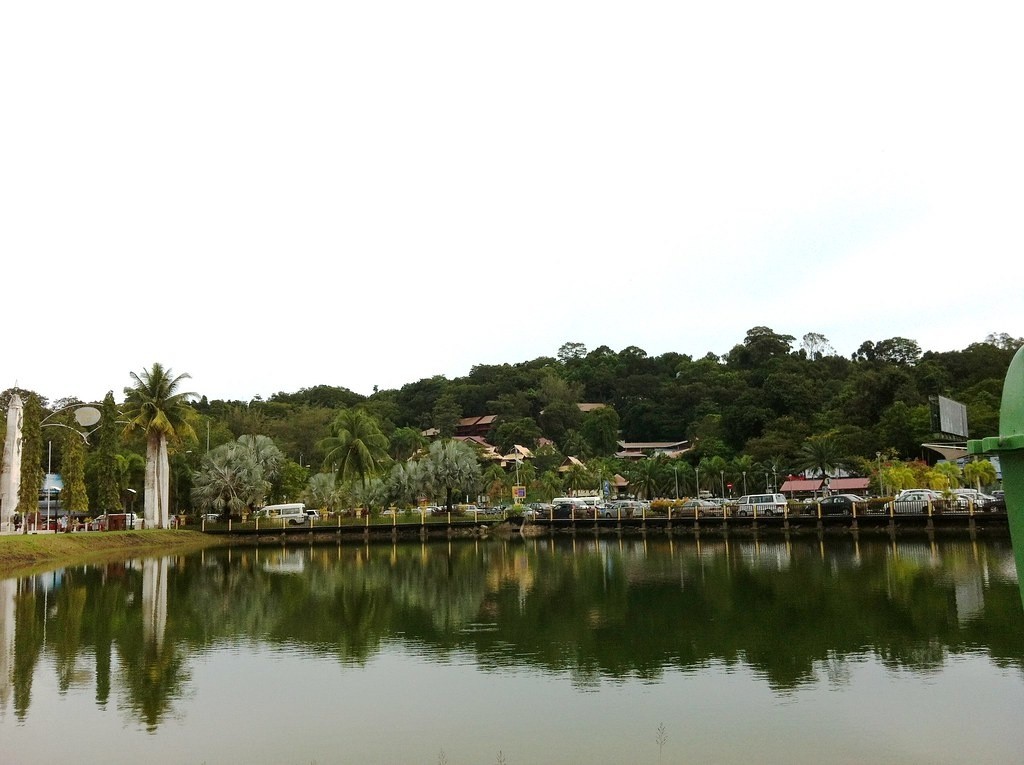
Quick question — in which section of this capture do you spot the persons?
[57,513,94,532]
[14,514,20,532]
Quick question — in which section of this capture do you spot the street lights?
[719,469,726,497]
[672,466,680,499]
[742,471,747,495]
[765,472,769,493]
[694,466,702,500]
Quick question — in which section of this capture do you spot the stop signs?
[727,482,733,489]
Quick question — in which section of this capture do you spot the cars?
[672,497,737,516]
[201,513,222,525]
[77,522,92,530]
[597,500,653,517]
[951,489,1005,513]
[531,502,553,516]
[378,503,504,520]
[804,493,868,515]
[42,519,58,530]
[306,509,320,520]
[882,489,944,514]
[504,506,539,519]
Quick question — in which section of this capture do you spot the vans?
[551,497,590,511]
[737,493,788,516]
[253,503,305,524]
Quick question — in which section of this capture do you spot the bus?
[577,496,602,510]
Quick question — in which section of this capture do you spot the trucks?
[92,514,137,529]
